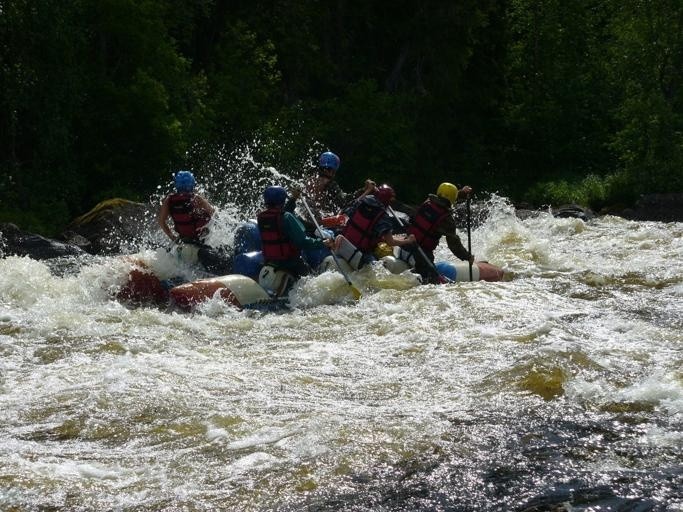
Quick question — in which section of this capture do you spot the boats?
[118,219,509,313]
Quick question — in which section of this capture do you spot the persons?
[157,170,220,267]
[342,182,417,265]
[258,180,344,274]
[293,149,376,230]
[403,182,474,271]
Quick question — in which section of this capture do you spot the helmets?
[262,185,289,205]
[317,150,341,171]
[437,181,458,204]
[373,184,397,203]
[173,169,197,189]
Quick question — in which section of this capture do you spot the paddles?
[365,180,456,284]
[299,192,363,300]
